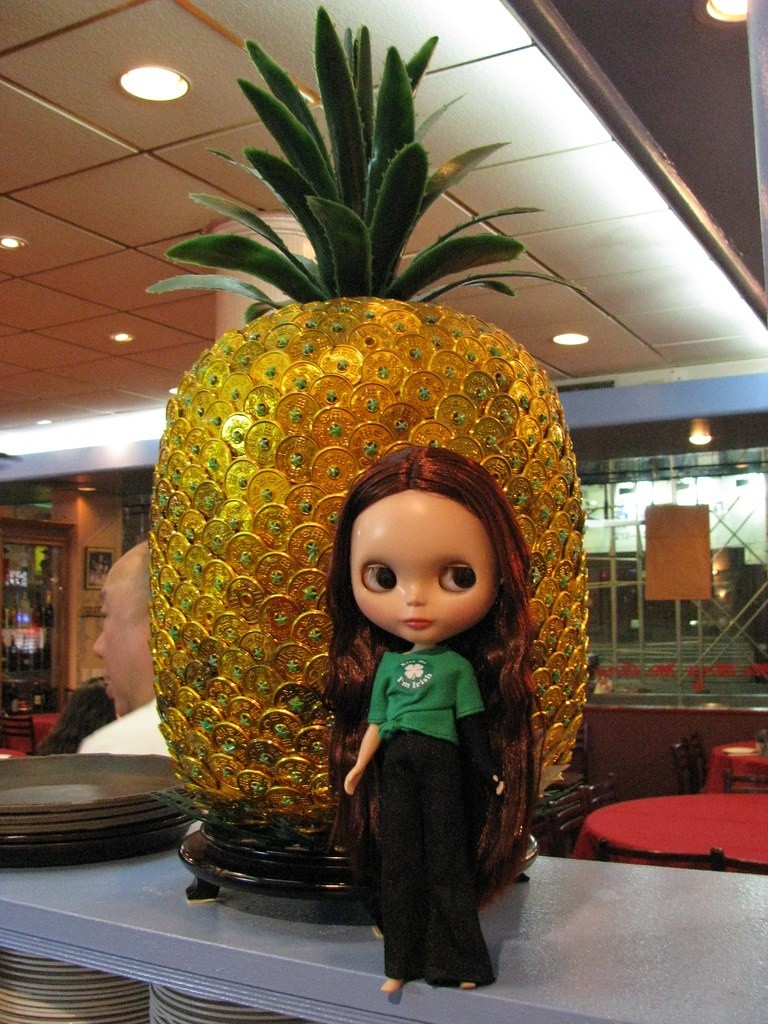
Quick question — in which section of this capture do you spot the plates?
[0,946,307,1024]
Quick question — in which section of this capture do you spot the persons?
[324,446,536,993]
[35,543,171,758]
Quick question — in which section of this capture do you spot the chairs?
[1,715,36,754]
[722,768,768,794]
[672,732,705,796]
[532,723,620,856]
[596,838,726,872]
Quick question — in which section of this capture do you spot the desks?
[574,796,766,865]
[703,741,767,795]
[4,713,61,754]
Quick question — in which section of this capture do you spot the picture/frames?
[86,547,115,591]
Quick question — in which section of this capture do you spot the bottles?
[0,565,54,715]
